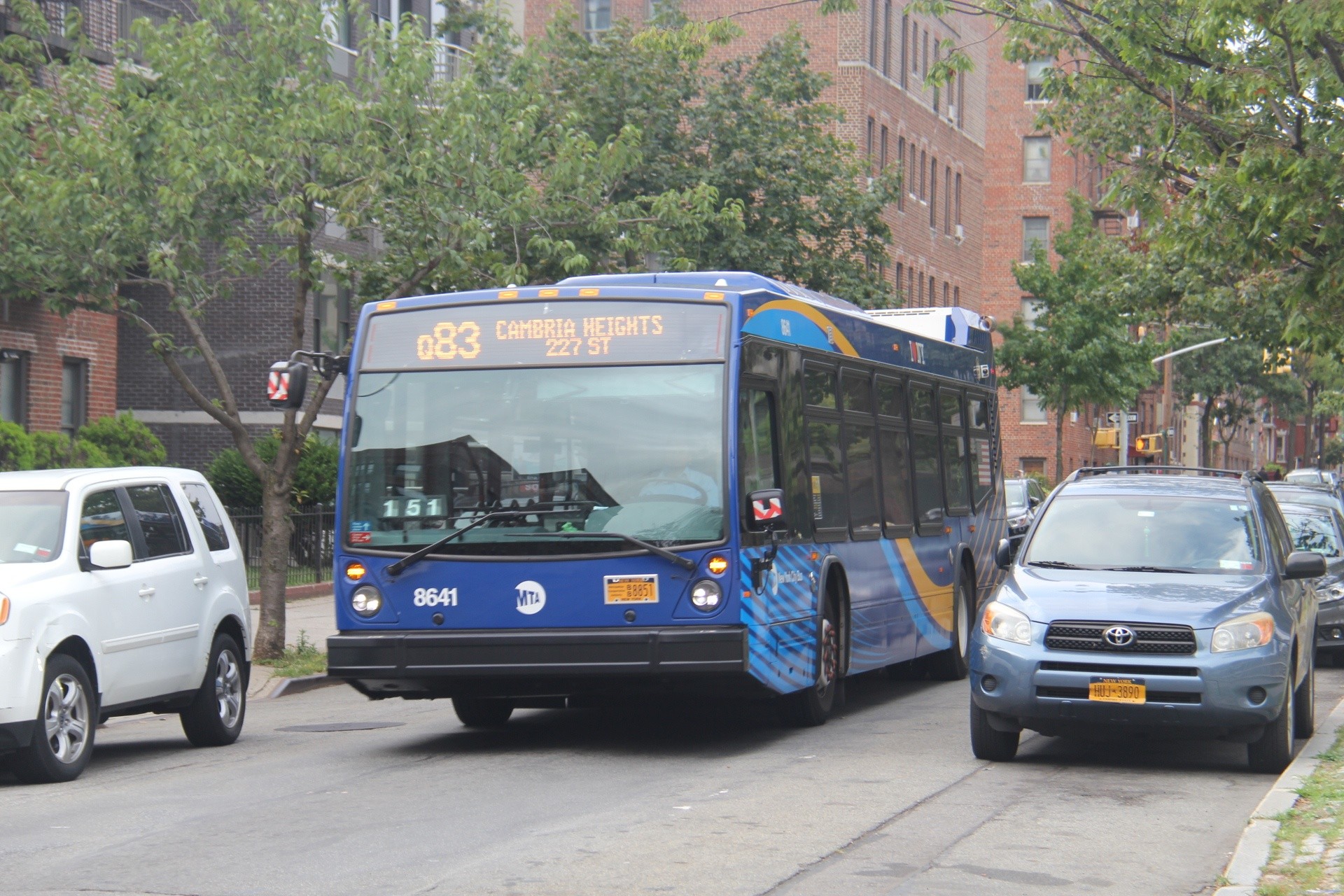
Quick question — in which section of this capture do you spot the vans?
[2,468,252,782]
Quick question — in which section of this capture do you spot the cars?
[1003,477,1046,550]
[1323,471,1342,487]
[1259,481,1344,655]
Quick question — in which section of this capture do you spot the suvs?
[1283,469,1325,485]
[970,465,1327,775]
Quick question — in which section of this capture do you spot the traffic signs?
[1105,412,1141,425]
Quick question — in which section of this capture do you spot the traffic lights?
[1263,346,1292,376]
[1133,436,1156,453]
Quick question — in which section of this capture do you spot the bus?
[265,271,1006,731]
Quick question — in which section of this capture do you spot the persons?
[641,436,721,508]
